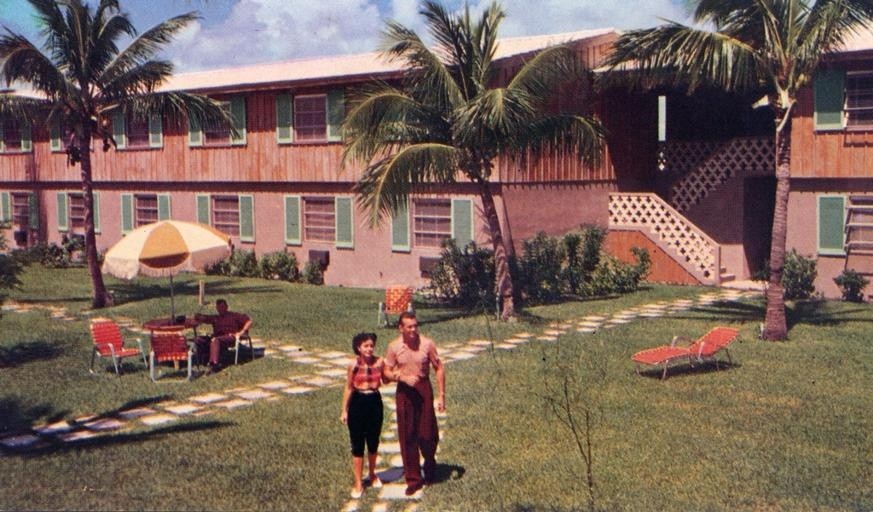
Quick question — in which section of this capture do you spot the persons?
[337,332,403,500]
[191,298,252,375]
[381,311,446,497]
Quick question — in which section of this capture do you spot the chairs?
[89,317,148,376]
[377,285,416,327]
[631,326,740,380]
[221,313,254,365]
[149,329,199,381]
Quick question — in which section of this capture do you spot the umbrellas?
[99,218,232,321]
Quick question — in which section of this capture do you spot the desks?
[143,318,202,371]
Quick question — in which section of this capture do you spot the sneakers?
[405,461,436,496]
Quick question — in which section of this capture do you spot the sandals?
[348,473,384,500]
